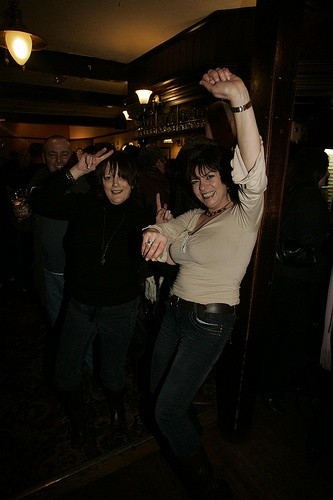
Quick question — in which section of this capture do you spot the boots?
[55,391,96,449]
[100,385,129,450]
[187,446,234,500]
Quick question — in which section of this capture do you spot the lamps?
[0,0,48,73]
[120,85,160,122]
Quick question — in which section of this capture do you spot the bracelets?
[66,169,77,184]
[231,100,253,113]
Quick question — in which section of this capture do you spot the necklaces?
[100,204,126,266]
[205,200,232,216]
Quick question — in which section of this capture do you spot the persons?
[13,134,94,377]
[142,65,270,500]
[263,143,330,373]
[30,147,174,447]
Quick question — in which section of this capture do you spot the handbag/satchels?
[273,239,314,268]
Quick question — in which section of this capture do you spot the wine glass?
[11,188,31,220]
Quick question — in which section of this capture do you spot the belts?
[170,294,234,314]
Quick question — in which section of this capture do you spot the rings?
[147,240,153,245]
